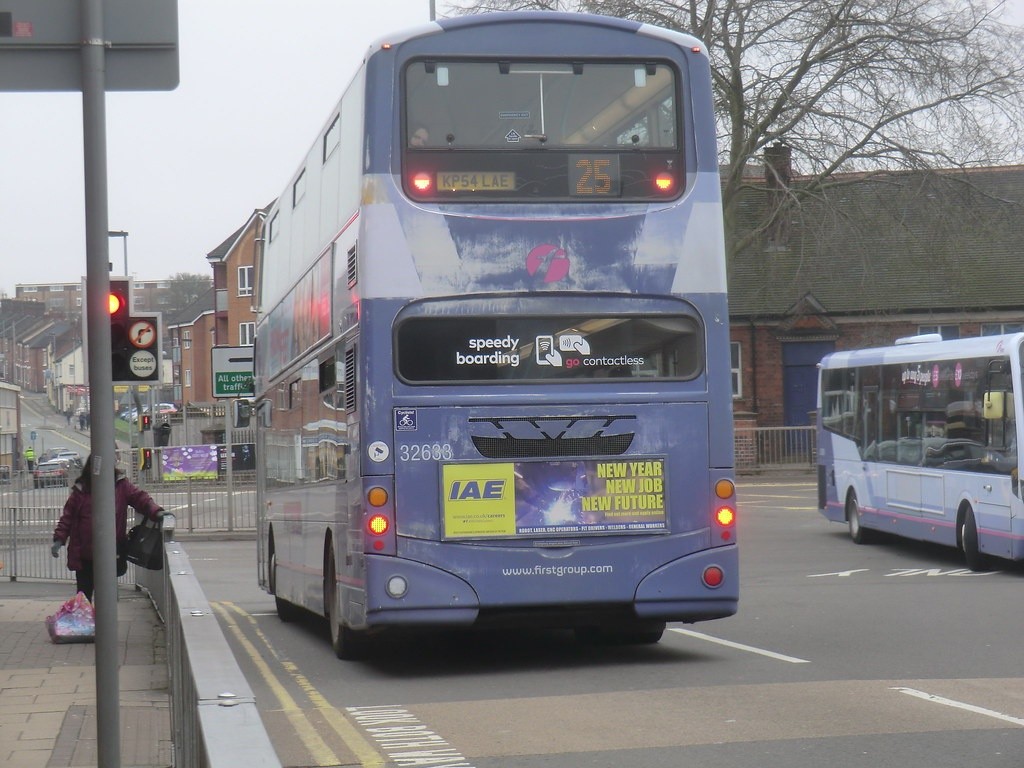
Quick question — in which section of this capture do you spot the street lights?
[107,230,132,448]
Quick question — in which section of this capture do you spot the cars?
[863,438,1018,484]
[33,448,85,489]
[121,403,177,425]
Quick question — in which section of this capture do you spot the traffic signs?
[212,345,254,398]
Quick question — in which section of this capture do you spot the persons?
[67,408,72,425]
[408,122,429,148]
[79,412,90,430]
[24,447,36,474]
[51,454,175,603]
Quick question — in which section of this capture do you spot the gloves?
[51,539,62,558]
[155,509,174,518]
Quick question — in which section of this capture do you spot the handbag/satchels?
[126,501,163,569]
[45,591,96,644]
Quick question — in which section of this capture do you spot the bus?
[816,334,1024,572]
[233,11,741,662]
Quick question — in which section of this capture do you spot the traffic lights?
[83,275,130,387]
[139,415,151,432]
[140,448,152,471]
